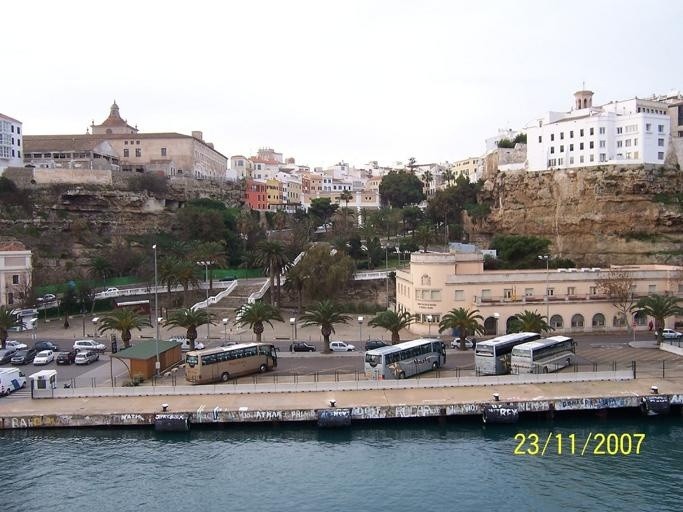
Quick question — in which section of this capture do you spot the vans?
[0,368,26,396]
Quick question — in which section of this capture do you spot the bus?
[185,343,280,385]
[364,338,449,380]
[475,332,577,375]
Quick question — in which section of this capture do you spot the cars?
[655,329,683,339]
[219,276,235,282]
[57,339,107,365]
[365,339,386,351]
[0,340,57,365]
[451,337,473,349]
[330,341,355,351]
[289,341,316,352]
[44,294,56,302]
[221,341,238,346]
[170,337,205,350]
[105,288,119,294]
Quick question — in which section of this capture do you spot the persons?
[648,319,653,331]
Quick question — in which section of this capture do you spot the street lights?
[494,313,500,336]
[290,317,296,341]
[152,244,159,379]
[426,315,432,334]
[197,261,216,339]
[538,255,550,337]
[358,316,363,340]
[223,318,229,341]
